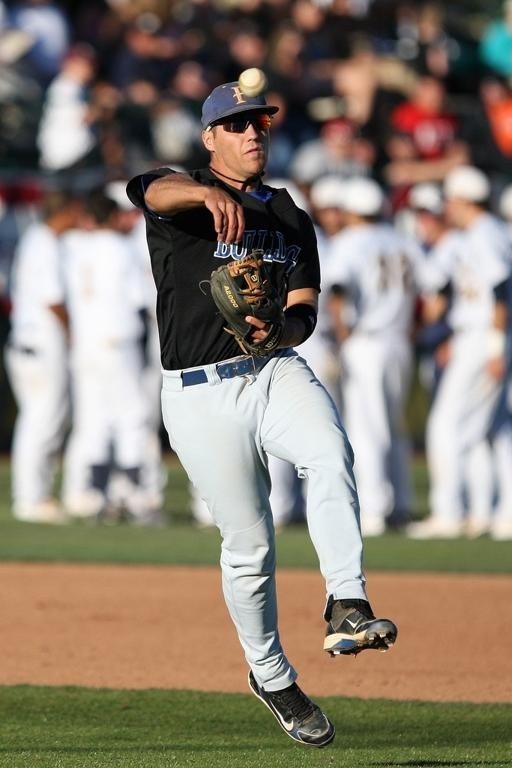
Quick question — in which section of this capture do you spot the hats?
[200,81,281,128]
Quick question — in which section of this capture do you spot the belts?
[182,345,297,387]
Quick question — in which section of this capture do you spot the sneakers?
[247,669,336,746]
[322,597,399,657]
[11,481,172,529]
[359,503,512,540]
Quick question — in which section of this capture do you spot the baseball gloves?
[211,248,286,360]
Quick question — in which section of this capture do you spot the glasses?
[205,110,274,133]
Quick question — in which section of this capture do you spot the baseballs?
[238,68,269,97]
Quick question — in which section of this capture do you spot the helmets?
[299,66,512,220]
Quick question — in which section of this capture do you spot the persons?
[124,80,398,750]
[0,2,511,542]
